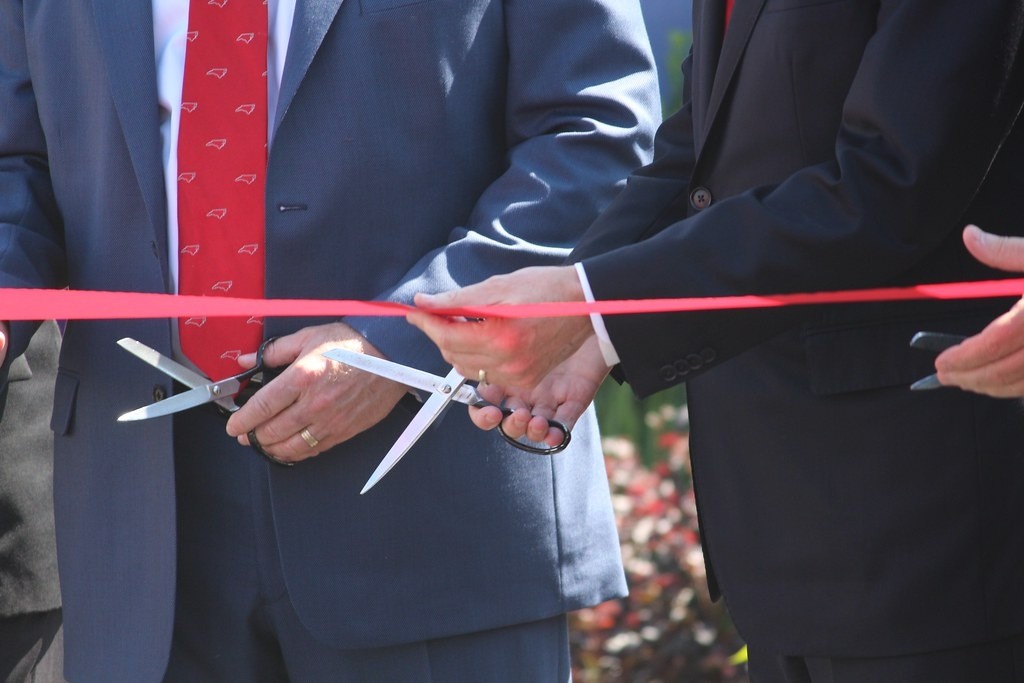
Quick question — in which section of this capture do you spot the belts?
[172,380,262,415]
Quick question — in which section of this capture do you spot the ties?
[176,0,270,399]
[724,0,735,36]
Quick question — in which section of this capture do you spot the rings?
[299,428,318,447]
[478,369,490,388]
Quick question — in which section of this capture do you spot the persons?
[406,0,1024,683]
[0,0,663,683]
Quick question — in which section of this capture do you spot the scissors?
[320,338,571,497]
[909,330,972,393]
[113,334,311,468]
